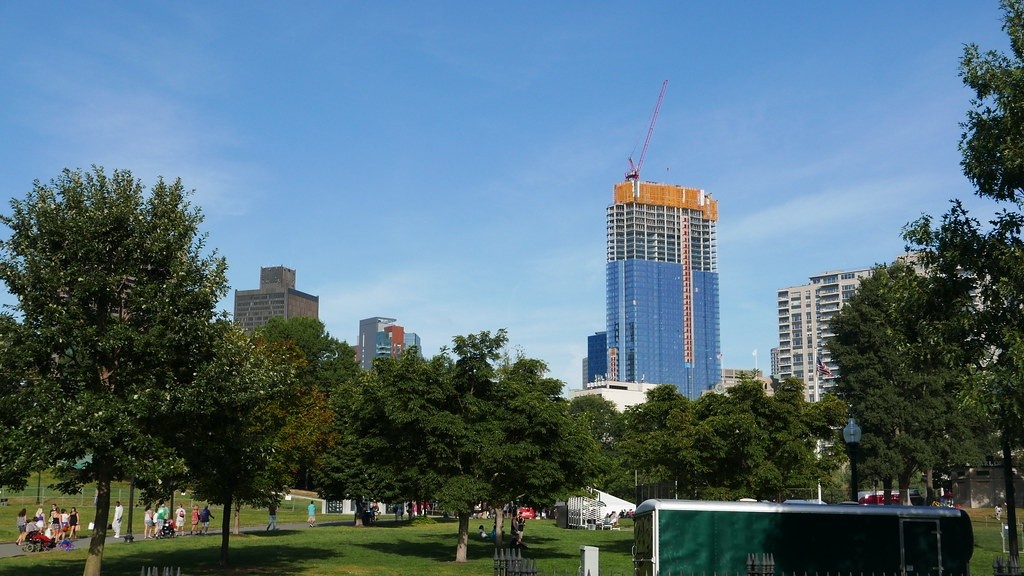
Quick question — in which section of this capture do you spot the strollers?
[161,521,177,538]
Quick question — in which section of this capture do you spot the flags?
[816,356,832,375]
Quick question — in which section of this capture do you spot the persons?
[199,505,214,535]
[266,504,279,532]
[191,505,201,535]
[175,504,187,536]
[112,501,123,538]
[49,507,62,545]
[472,504,531,553]
[994,501,1008,521]
[26,517,56,551]
[15,508,27,545]
[48,503,59,525]
[307,500,316,527]
[142,497,174,539]
[603,508,635,527]
[35,507,47,534]
[362,496,431,520]
[67,506,80,539]
[58,509,70,542]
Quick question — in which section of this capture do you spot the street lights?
[844,416,863,503]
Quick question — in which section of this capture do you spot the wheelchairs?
[22,534,40,552]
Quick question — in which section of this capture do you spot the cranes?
[626,78,668,181]
